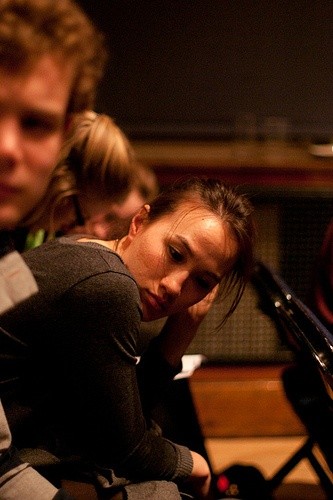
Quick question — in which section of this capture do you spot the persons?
[0,0,253,500]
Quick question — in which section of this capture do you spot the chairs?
[243,257,333,500]
